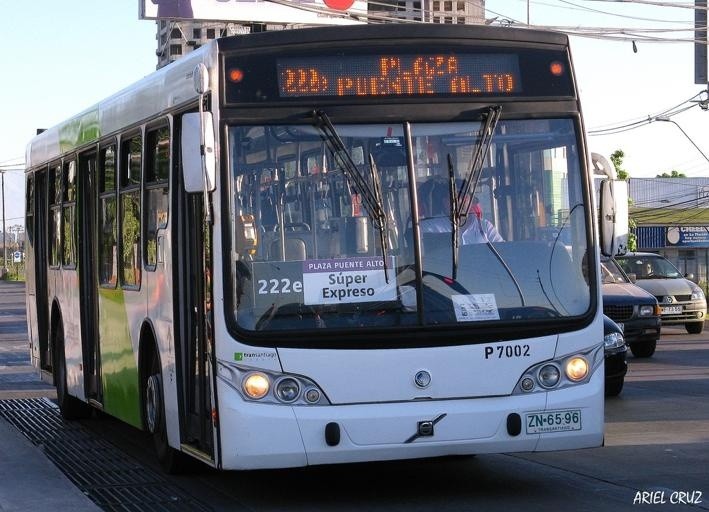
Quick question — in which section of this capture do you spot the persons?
[640,262,657,276]
[419,179,507,246]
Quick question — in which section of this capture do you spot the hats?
[435,180,478,201]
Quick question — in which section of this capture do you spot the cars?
[594,240,709,396]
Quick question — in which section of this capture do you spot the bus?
[19,18,611,480]
